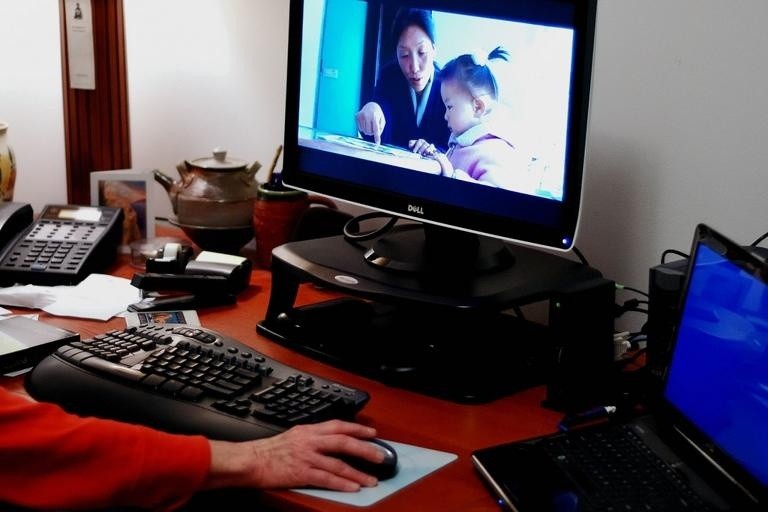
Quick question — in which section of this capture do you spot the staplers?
[127,272,236,312]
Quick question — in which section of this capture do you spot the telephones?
[0,202,124,285]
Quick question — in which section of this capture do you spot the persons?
[355,9,448,157]
[1,388,388,511]
[428,43,522,191]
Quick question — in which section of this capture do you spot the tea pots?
[153,149,260,228]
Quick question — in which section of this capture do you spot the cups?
[249,171,340,269]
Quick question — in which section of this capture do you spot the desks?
[0,227,644,512]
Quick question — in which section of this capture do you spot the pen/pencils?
[578,406,615,420]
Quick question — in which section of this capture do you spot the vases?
[0,122,17,203]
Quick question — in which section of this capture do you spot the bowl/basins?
[151,216,255,253]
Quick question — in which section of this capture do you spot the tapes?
[154,243,181,262]
